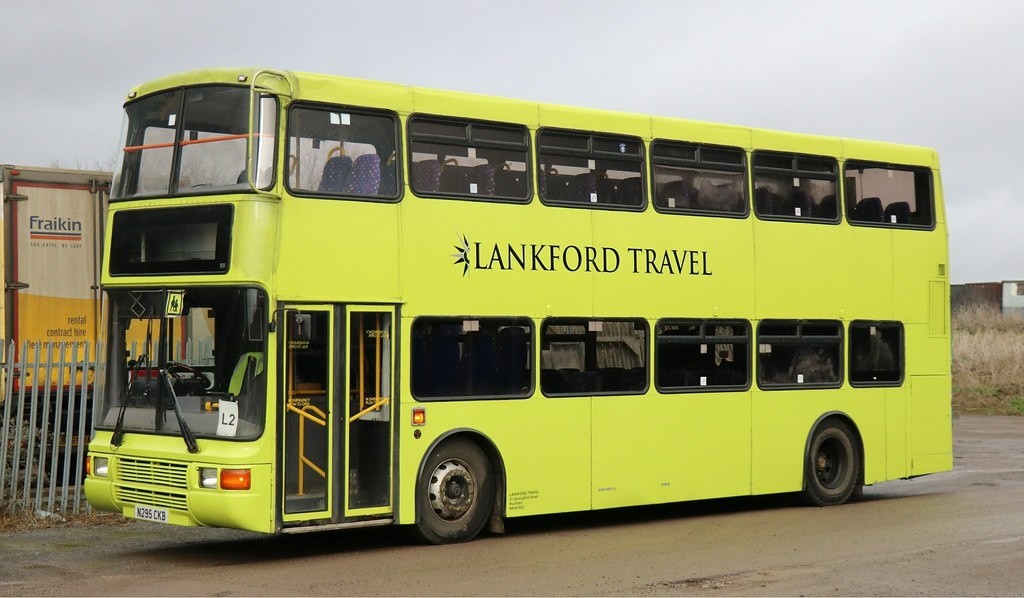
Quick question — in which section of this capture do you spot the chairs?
[411,328,894,399]
[316,154,911,229]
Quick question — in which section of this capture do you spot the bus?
[83,66,952,544]
